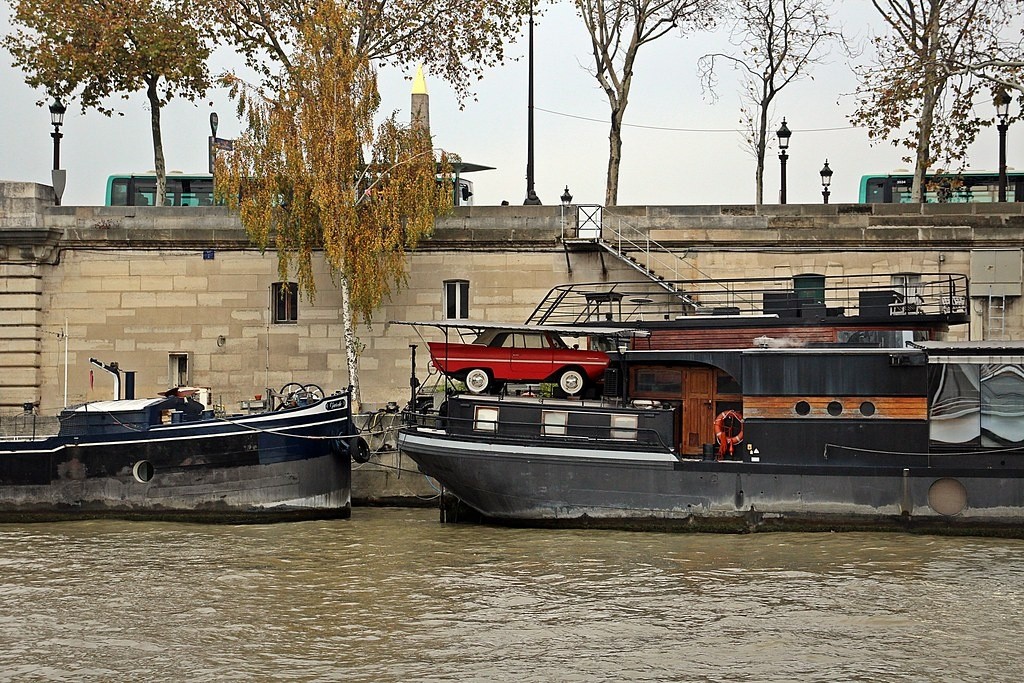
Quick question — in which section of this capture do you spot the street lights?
[776,116,792,204]
[48,95,67,206]
[993,88,1012,203]
[819,158,833,204]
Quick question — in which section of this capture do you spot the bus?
[105,170,475,206]
[859,169,1024,204]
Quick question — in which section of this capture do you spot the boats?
[389,320,1024,534]
[0,317,352,524]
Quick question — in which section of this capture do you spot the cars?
[427,328,611,396]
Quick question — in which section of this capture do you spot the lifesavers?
[349,437,370,463]
[714,409,744,444]
